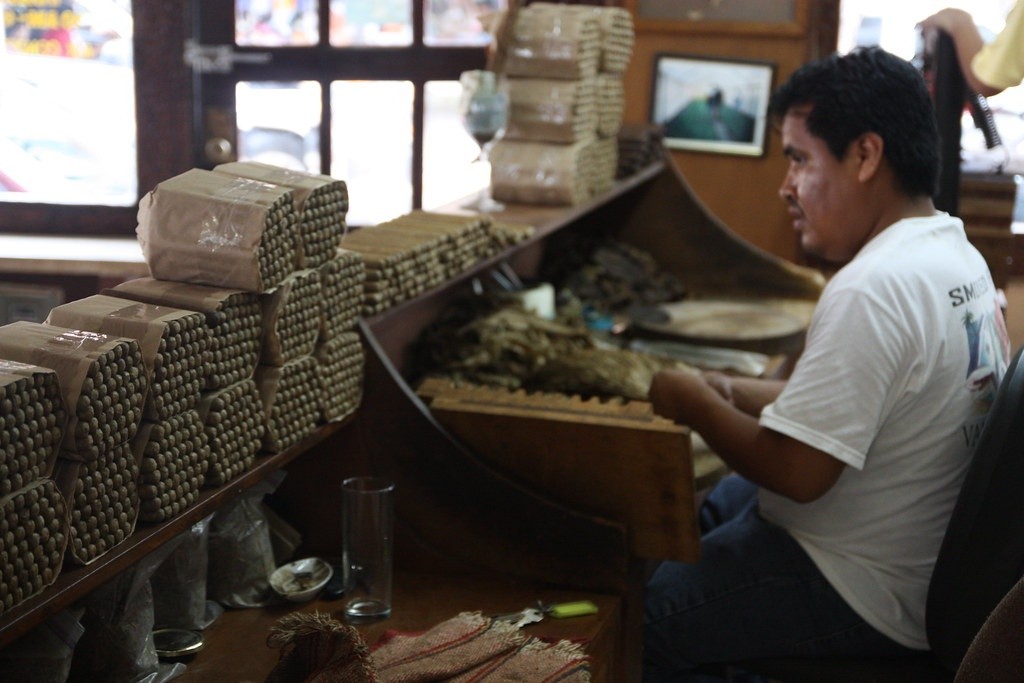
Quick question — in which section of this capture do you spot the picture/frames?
[647,52,778,159]
[626,0,811,37]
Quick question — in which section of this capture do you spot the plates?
[638,294,814,341]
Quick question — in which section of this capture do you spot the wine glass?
[453,68,511,214]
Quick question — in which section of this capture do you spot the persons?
[625,47,1016,683]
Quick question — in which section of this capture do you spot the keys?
[492,603,544,630]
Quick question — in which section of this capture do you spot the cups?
[339,474,397,626]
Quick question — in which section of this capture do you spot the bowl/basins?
[268,556,335,603]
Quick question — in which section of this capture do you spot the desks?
[0,140,827,683]
